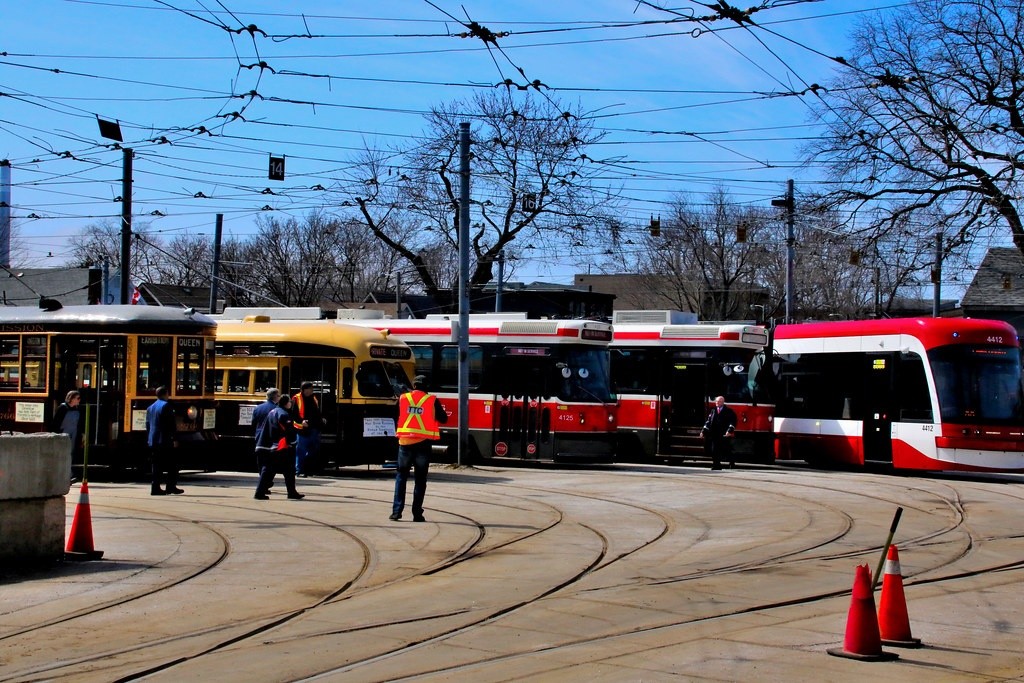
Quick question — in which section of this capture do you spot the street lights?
[770,178,794,326]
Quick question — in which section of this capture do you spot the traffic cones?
[873,545,924,649]
[825,563,902,662]
[64,481,107,561]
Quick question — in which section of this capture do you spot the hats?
[413,375,427,385]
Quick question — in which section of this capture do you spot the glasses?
[74,397,81,400]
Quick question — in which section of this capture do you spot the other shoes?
[166,487,184,494]
[412,515,425,522]
[70,477,77,484]
[388,512,402,519]
[255,493,269,500]
[288,491,305,499]
[151,488,167,495]
[265,490,271,495]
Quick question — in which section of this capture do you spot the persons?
[699,396,738,471]
[53,391,86,485]
[290,380,327,477]
[253,395,305,500]
[251,388,280,495]
[389,375,448,522]
[145,386,185,495]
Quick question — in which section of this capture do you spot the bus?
[592,308,776,462]
[0,300,220,480]
[335,309,621,466]
[113,307,417,478]
[759,316,1024,475]
